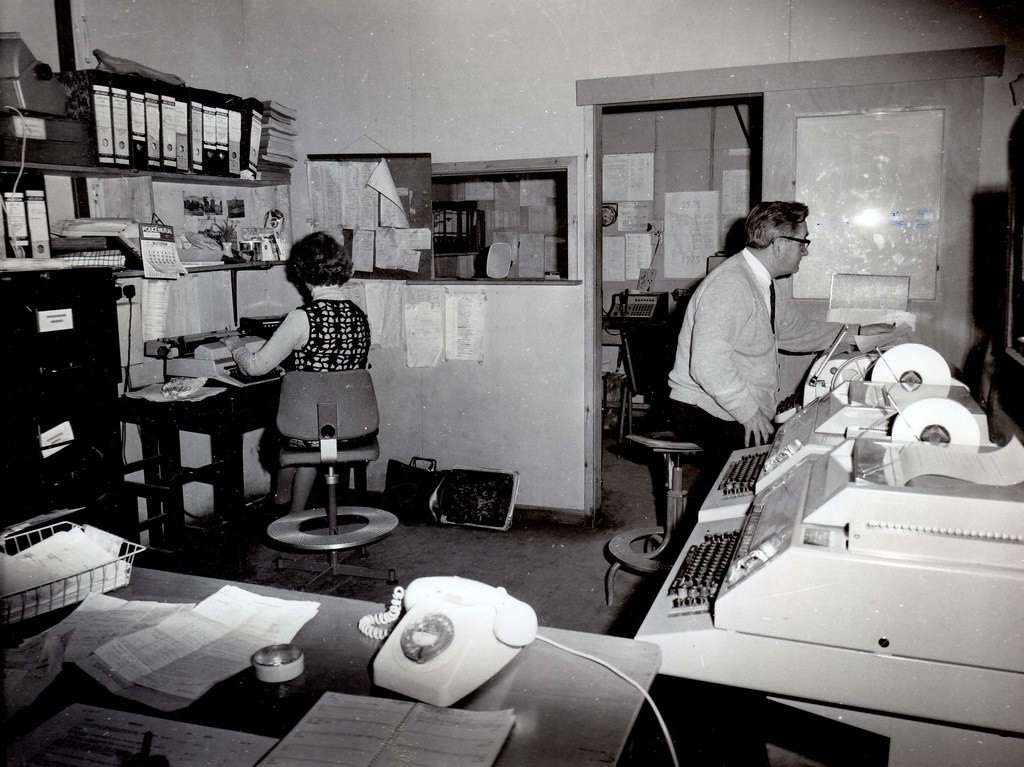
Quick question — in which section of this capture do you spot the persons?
[218,230,372,532]
[667,201,896,543]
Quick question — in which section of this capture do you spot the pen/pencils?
[211,215,238,236]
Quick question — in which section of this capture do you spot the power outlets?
[644,220,664,232]
[115,277,140,305]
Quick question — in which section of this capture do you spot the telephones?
[371,574,539,709]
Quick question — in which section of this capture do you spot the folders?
[83,68,263,180]
[4,190,50,258]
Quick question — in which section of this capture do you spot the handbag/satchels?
[381,456,450,525]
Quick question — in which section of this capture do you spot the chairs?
[267,371,401,586]
[606,334,702,607]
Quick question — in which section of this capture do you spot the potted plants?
[213,220,238,257]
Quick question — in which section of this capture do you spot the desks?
[118,382,287,557]
[0,567,662,767]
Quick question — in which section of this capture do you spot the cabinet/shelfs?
[0,161,292,276]
[0,268,131,557]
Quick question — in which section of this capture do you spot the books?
[256,100,298,181]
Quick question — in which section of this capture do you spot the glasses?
[772,235,811,252]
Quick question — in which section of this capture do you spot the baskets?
[0,521,147,626]
